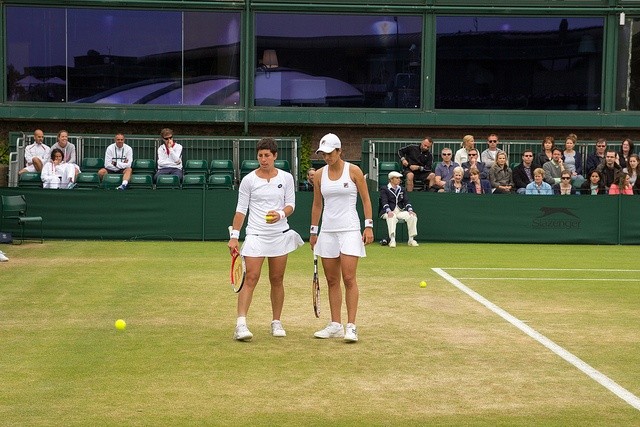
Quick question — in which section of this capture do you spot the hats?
[388,171,403,179]
[315,132,341,155]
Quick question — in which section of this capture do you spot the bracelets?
[309,225,319,233]
[418,165,421,170]
[114,162,116,166]
[229,229,240,239]
[275,210,285,219]
[365,218,373,227]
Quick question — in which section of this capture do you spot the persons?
[299,167,316,191]
[227,136,305,340]
[481,133,509,169]
[467,166,492,194]
[561,132,585,190]
[596,149,623,193]
[461,148,487,184]
[552,169,576,195]
[378,170,419,247]
[580,170,606,194]
[536,136,556,168]
[443,166,469,193]
[18,129,51,175]
[434,147,460,192]
[512,149,542,194]
[40,148,76,189]
[398,136,435,192]
[309,132,374,341]
[542,145,571,188]
[525,167,554,195]
[98,133,133,190]
[152,127,183,189]
[454,134,481,166]
[489,151,517,193]
[618,138,634,168]
[44,129,81,187]
[585,137,607,179]
[623,152,640,194]
[608,168,634,194]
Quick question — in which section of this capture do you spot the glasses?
[596,144,606,147]
[442,152,452,156]
[561,176,570,180]
[605,155,615,158]
[488,139,498,144]
[523,154,533,157]
[162,135,173,140]
[468,153,476,156]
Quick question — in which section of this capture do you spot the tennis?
[116,319,126,330]
[420,281,426,287]
[266,214,274,222]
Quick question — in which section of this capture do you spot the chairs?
[75,173,101,190]
[1,195,43,244]
[432,161,443,172]
[102,174,123,190]
[17,172,43,189]
[379,174,388,188]
[377,198,409,243]
[182,174,207,190]
[127,175,153,190]
[131,159,156,175]
[378,162,401,173]
[510,162,521,169]
[182,160,208,175]
[401,160,427,185]
[240,160,291,181]
[80,158,104,172]
[210,160,234,181]
[207,174,235,190]
[155,175,180,190]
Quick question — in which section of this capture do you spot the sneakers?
[313,322,345,339]
[234,324,253,341]
[118,184,126,191]
[344,323,358,343]
[0,250,10,262]
[271,319,286,337]
[389,241,396,248]
[407,239,420,247]
